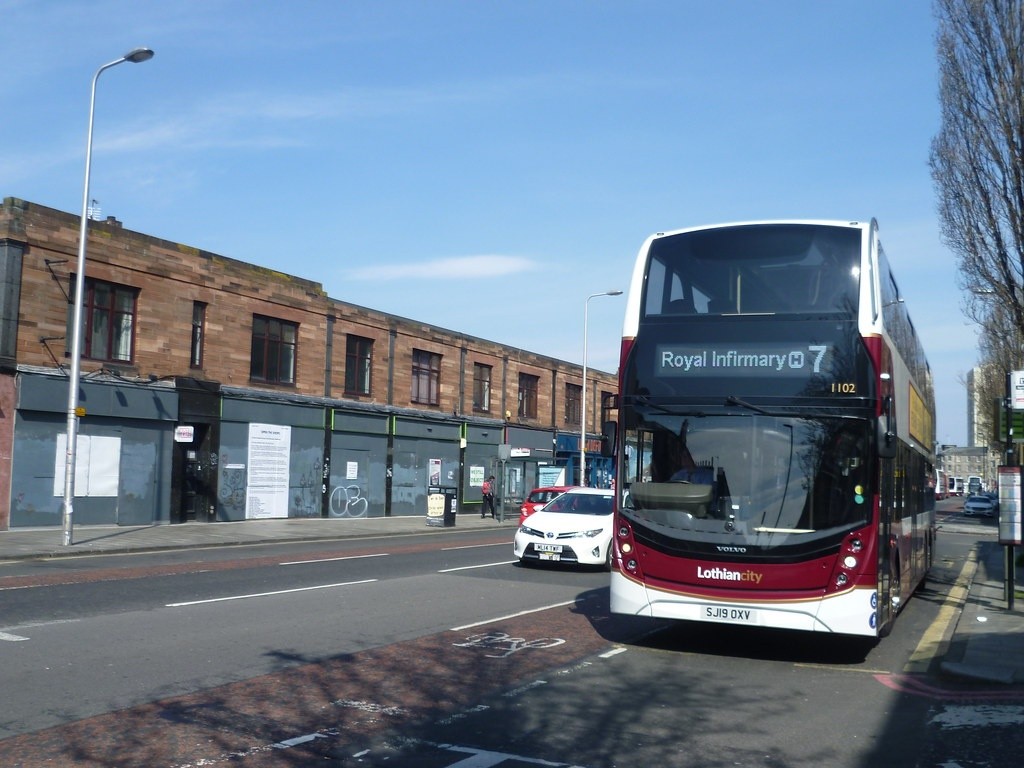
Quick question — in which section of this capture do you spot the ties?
[687,471,693,482]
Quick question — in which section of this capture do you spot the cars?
[512,486,628,573]
[963,495,996,519]
[982,492,1000,511]
[519,485,578,528]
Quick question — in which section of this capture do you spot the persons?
[662,445,719,512]
[608,478,616,490]
[481,476,495,518]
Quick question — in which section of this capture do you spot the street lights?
[580,289,625,488]
[973,286,1014,465]
[62,47,157,546]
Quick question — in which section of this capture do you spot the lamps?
[82,369,158,385]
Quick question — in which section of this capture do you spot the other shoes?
[481,515,485,518]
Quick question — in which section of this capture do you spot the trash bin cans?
[426,485,458,527]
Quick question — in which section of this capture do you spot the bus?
[948,475,964,497]
[598,215,940,639]
[934,467,949,502]
[966,476,983,495]
